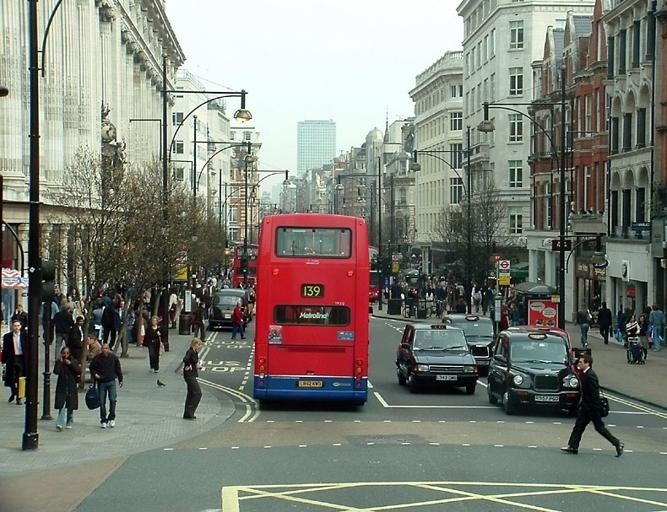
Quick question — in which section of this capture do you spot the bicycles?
[403,300,444,321]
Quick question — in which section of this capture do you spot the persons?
[574,300,666,365]
[1,275,256,364]
[391,274,527,343]
[53,347,82,432]
[142,315,164,373]
[183,338,202,420]
[560,354,624,458]
[1,320,28,405]
[89,343,123,429]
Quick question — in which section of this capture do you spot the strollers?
[627,333,647,367]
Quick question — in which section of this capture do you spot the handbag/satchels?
[86,387,100,409]
[600,397,609,417]
[610,327,613,337]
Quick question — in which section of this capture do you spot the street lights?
[130,89,252,350]
[335,173,386,315]
[478,100,600,331]
[169,138,261,347]
[216,167,293,288]
[408,147,493,320]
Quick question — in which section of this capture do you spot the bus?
[252,210,371,406]
[231,245,259,288]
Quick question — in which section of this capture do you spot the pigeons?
[157,379,166,388]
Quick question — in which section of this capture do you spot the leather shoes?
[560,446,578,454]
[9,395,14,402]
[16,396,22,404]
[615,442,624,457]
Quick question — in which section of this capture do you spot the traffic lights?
[39,259,57,303]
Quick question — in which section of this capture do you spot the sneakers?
[110,419,115,428]
[101,422,106,428]
[65,426,72,429]
[56,425,63,431]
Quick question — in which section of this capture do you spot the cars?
[210,291,245,332]
[396,322,478,395]
[488,328,583,416]
[445,315,498,365]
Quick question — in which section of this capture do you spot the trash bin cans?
[179,315,192,336]
[389,299,401,315]
